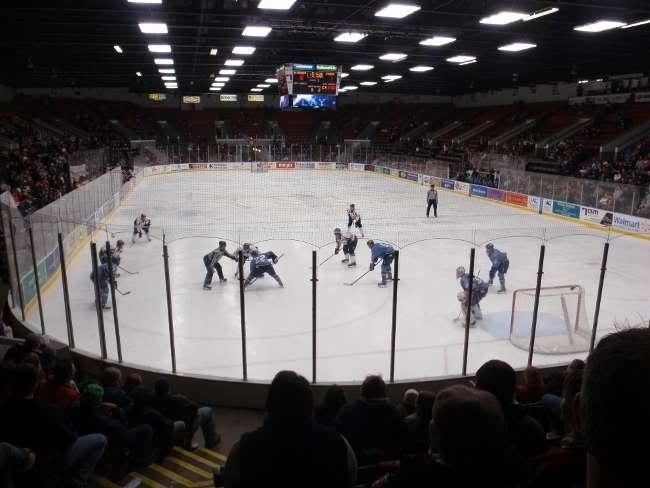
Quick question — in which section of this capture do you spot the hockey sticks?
[118,266,139,275]
[453,270,482,321]
[115,287,130,295]
[342,259,383,286]
[309,248,343,271]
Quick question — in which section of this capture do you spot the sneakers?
[101,305,112,312]
[205,431,221,449]
[234,273,239,278]
[342,259,357,268]
[190,442,200,452]
[219,277,227,283]
[203,284,212,290]
[377,277,393,288]
[460,315,483,328]
[486,279,507,294]
[279,282,285,288]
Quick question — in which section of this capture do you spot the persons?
[98,240,123,263]
[333,229,360,269]
[424,184,440,218]
[131,214,153,243]
[454,267,490,329]
[0,333,222,487]
[484,243,509,294]
[449,100,650,218]
[347,203,366,235]
[242,250,283,288]
[0,110,137,220]
[368,241,394,286]
[138,130,449,169]
[232,242,261,280]
[202,241,237,289]
[221,329,649,487]
[89,255,120,309]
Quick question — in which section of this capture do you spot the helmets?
[243,242,259,255]
[367,239,374,248]
[350,203,355,209]
[486,243,494,249]
[455,266,466,275]
[112,255,121,265]
[333,228,341,234]
[219,241,226,247]
[116,240,125,246]
[141,213,147,217]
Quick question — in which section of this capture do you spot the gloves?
[271,254,279,264]
[138,231,142,238]
[334,248,339,255]
[369,263,375,271]
